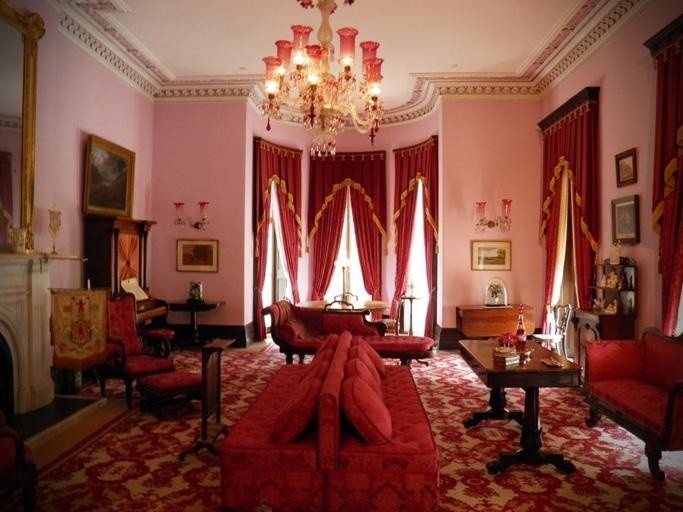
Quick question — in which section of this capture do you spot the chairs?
[0,413,42,512]
[531,301,574,364]
[97,293,179,410]
[376,298,404,337]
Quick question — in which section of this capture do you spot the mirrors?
[1,0,45,250]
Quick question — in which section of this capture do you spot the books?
[494,352,527,366]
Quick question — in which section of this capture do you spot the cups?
[8,226,27,254]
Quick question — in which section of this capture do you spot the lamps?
[173,201,211,232]
[256,0,384,156]
[473,199,513,232]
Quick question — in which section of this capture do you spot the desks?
[454,334,582,477]
[455,303,535,338]
[166,296,225,350]
[292,298,388,321]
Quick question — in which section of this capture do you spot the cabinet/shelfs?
[401,297,429,368]
[571,262,637,393]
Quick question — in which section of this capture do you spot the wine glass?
[47,206,64,256]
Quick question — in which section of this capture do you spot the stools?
[136,370,205,416]
[144,328,176,357]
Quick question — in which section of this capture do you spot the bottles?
[610,243,621,265]
[515,303,528,353]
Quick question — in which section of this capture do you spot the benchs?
[319,331,438,511]
[582,325,682,482]
[261,300,435,370]
[218,330,352,511]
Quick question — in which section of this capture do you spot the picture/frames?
[175,239,219,273]
[610,194,639,245]
[83,130,137,217]
[613,147,637,188]
[468,239,512,272]
